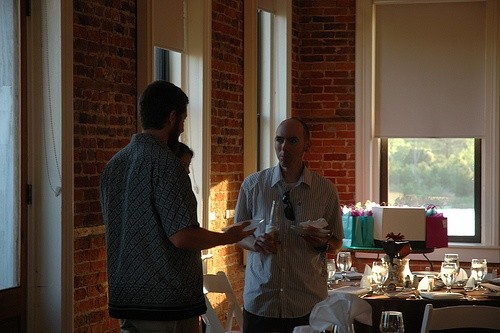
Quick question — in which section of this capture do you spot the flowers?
[385,232,405,241]
[340,199,381,216]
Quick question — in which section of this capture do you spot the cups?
[417,275,433,293]
[320,324,334,333]
[379,312,387,333]
[444,253,460,278]
[333,322,355,333]
[384,311,404,333]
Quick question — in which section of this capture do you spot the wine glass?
[441,262,456,293]
[471,259,487,291]
[325,259,336,292]
[336,251,352,283]
[372,262,388,294]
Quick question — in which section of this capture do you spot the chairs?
[420,303,500,333]
[200,271,244,333]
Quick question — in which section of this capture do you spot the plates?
[412,270,440,282]
[420,292,464,300]
[289,224,330,234]
[335,271,363,280]
[221,218,265,233]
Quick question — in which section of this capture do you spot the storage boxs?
[372,207,426,241]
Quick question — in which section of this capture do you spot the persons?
[234,117,344,333]
[100,80,257,333]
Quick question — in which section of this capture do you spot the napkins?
[456,268,468,283]
[466,275,475,289]
[361,264,373,288]
[310,292,371,333]
[418,275,429,291]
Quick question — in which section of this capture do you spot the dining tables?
[326,277,500,333]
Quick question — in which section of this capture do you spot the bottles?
[265,200,281,255]
[397,259,413,291]
